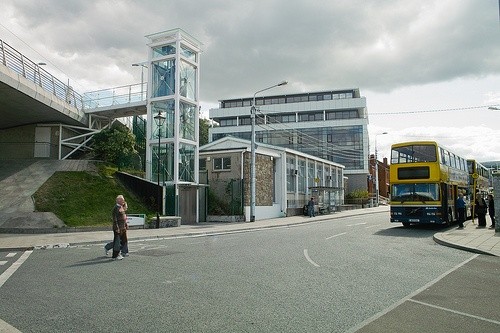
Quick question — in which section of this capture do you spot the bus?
[466,158,493,219]
[389,140,471,228]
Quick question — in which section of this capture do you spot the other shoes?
[490,225,494,229]
[105,247,108,255]
[121,252,130,256]
[113,255,123,260]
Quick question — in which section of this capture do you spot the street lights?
[249,80,289,223]
[132,62,148,101]
[375,131,388,208]
[153,109,167,227]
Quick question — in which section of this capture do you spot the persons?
[308,197,315,218]
[488,195,495,228]
[475,193,487,227]
[112,195,129,260]
[66,86,72,104]
[457,193,466,228]
